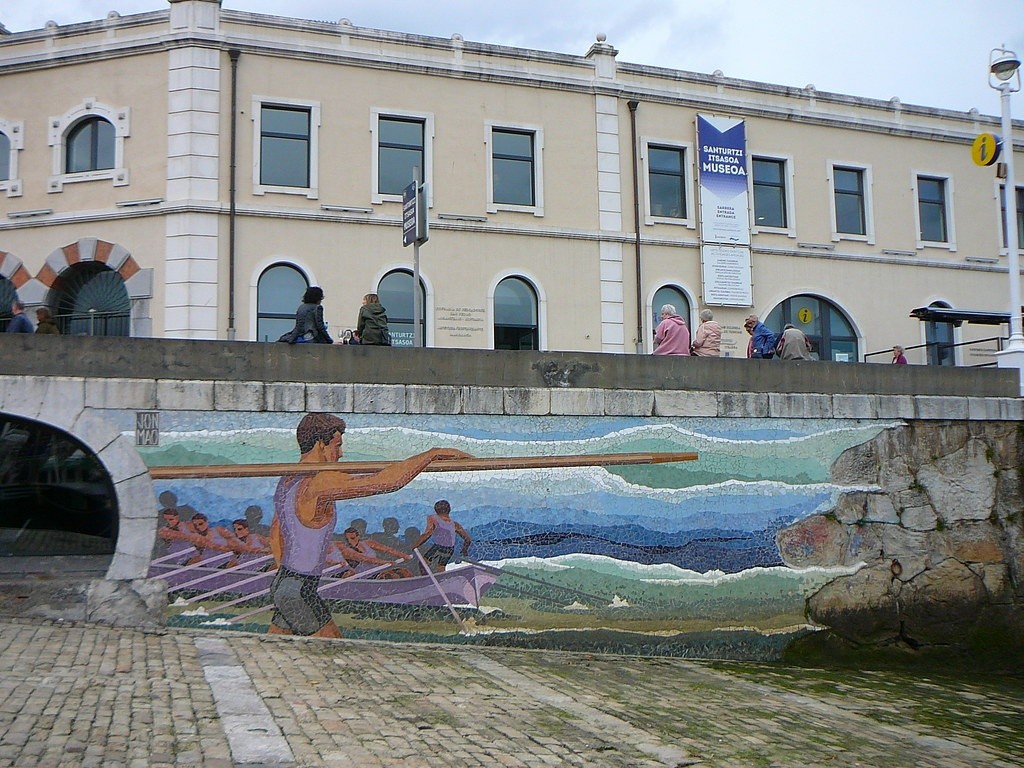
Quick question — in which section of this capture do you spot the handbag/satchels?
[343,329,359,345]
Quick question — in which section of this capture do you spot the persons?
[6,300,34,333]
[343,294,388,345]
[653,304,691,356]
[775,323,813,361]
[744,314,777,359]
[891,345,908,365]
[295,286,343,344]
[35,306,60,334]
[691,309,722,357]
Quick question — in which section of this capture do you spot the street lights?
[989,44,1024,367]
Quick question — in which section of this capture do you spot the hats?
[700,309,713,321]
[744,314,758,321]
[784,323,795,330]
